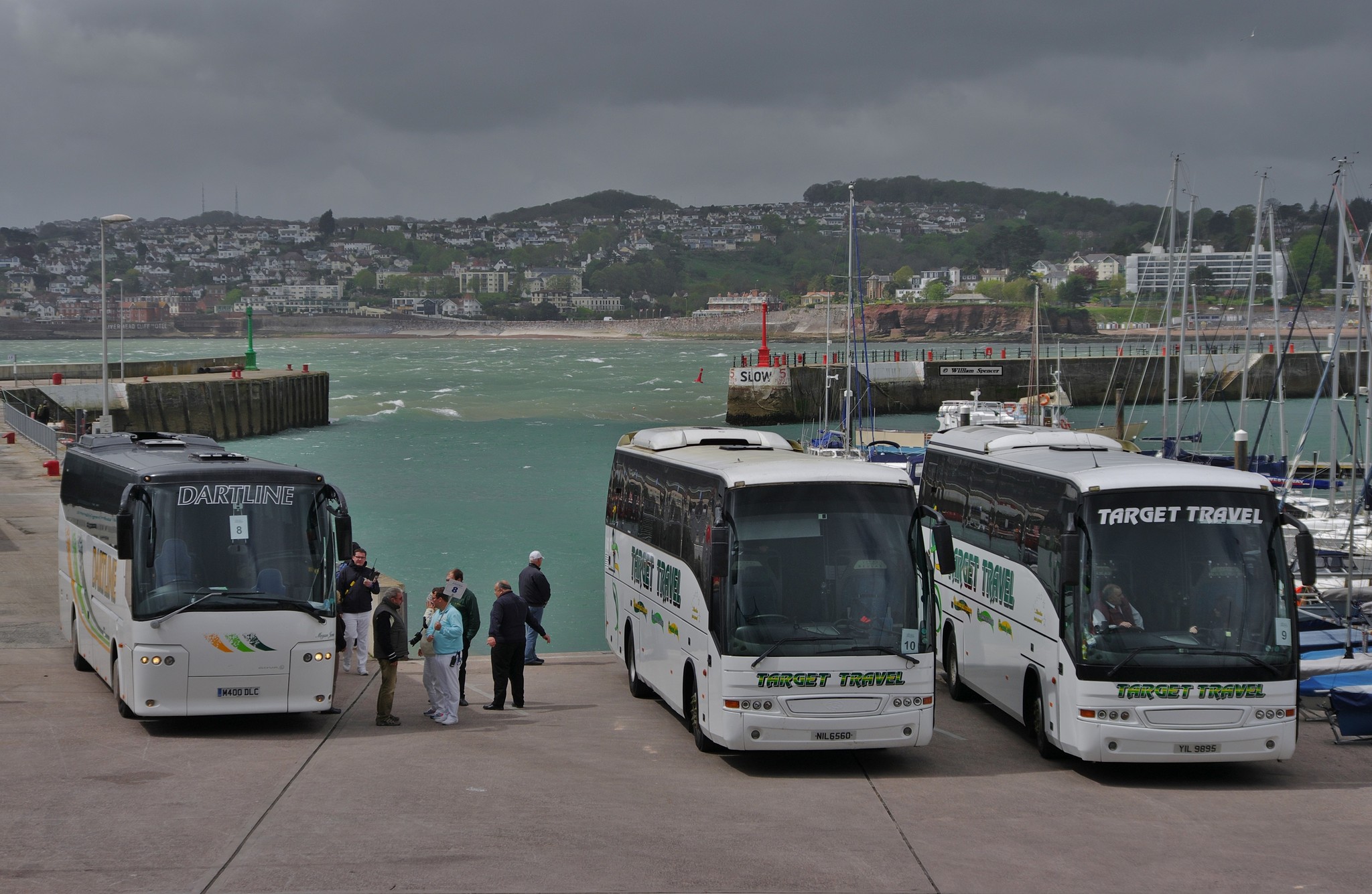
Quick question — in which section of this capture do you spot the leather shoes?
[524,661,542,665]
[460,699,468,706]
[512,702,523,708]
[321,707,341,714]
[536,658,544,662]
[483,704,503,710]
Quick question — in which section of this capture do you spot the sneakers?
[356,669,369,675]
[442,719,458,725]
[424,708,435,715]
[435,718,444,723]
[343,663,351,672]
[430,712,444,719]
[389,714,400,720]
[376,717,401,726]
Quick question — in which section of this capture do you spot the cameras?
[408,632,422,647]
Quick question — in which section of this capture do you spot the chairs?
[153,538,192,587]
[847,576,887,622]
[249,569,286,596]
[736,565,779,626]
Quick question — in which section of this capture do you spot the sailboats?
[806,184,873,463]
[1075,144,1372,745]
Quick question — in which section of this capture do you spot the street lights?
[111,278,125,384]
[99,214,134,414]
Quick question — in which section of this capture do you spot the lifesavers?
[1004,403,1016,414]
[1038,393,1050,405]
[1060,419,1068,429]
[1023,402,1027,414]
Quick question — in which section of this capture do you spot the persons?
[334,540,380,675]
[518,551,551,665]
[313,590,347,714]
[1185,591,1247,638]
[418,569,481,725]
[1091,583,1145,642]
[372,587,410,725]
[33,400,50,426]
[482,580,551,710]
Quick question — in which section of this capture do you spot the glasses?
[355,556,365,558]
[396,598,402,600]
[432,597,437,599]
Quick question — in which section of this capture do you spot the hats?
[427,591,434,603]
[529,550,545,559]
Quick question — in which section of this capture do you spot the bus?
[913,422,1321,767]
[599,426,956,758]
[55,428,356,721]
